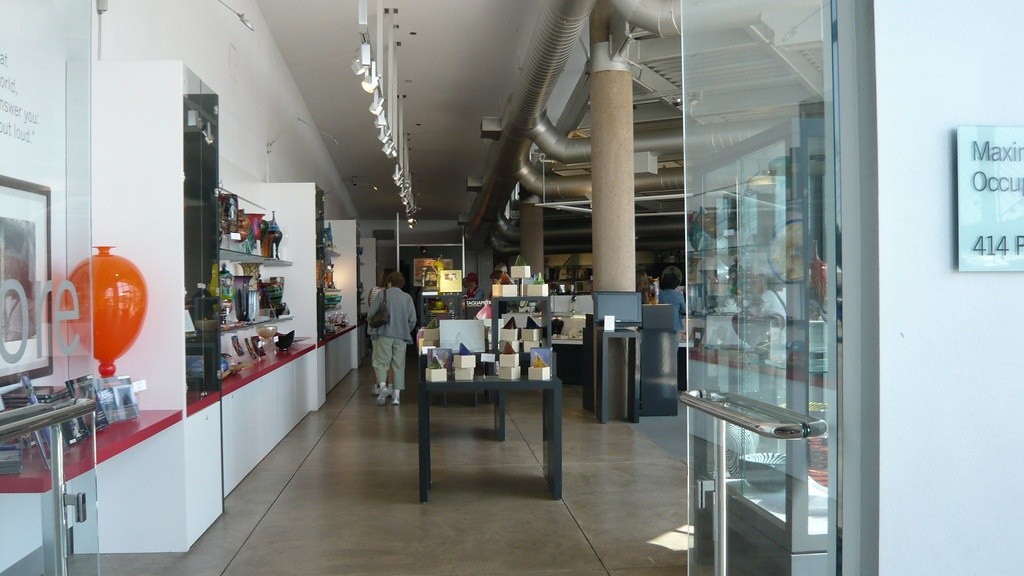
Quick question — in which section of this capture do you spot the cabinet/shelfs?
[219,185,294,333]
[688,180,803,329]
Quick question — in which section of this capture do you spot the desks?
[595,328,641,424]
[0,409,184,494]
[417,377,562,501]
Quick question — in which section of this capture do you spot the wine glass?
[256,326,278,358]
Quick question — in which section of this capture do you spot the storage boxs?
[423,265,551,382]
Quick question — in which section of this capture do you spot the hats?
[466,272,478,283]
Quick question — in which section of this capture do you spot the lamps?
[203,130,216,145]
[392,169,403,180]
[360,74,382,94]
[350,56,365,77]
[374,110,387,129]
[393,176,418,229]
[381,140,394,155]
[368,97,384,116]
[377,128,392,144]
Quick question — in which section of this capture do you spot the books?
[0,373,140,474]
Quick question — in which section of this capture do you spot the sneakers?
[390,390,401,405]
[376,384,390,407]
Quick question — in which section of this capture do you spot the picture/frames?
[0,174,54,388]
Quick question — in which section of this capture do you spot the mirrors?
[183,65,223,408]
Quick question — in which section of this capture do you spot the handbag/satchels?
[368,287,391,329]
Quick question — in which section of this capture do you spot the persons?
[747,271,786,391]
[636,270,651,304]
[462,272,485,319]
[489,263,515,319]
[367,268,417,406]
[659,273,686,352]
